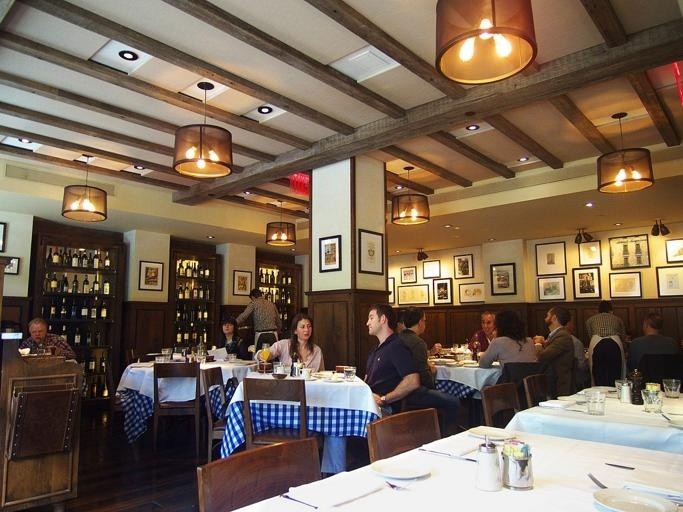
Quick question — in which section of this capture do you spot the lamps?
[659,219,670,236]
[582,229,593,241]
[172,81,233,182]
[650,220,659,237]
[436,1,539,87]
[391,167,430,225]
[59,155,109,224]
[417,249,422,261]
[573,229,582,244]
[596,112,655,196]
[420,248,428,260]
[264,199,297,247]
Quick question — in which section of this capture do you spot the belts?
[255,329,277,332]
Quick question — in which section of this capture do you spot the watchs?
[381,396,387,407]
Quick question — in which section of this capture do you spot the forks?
[660,413,673,422]
[385,480,403,490]
[589,472,611,489]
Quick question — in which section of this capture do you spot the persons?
[468,310,498,363]
[533,305,576,400]
[627,312,680,392]
[585,300,626,387]
[321,303,420,473]
[479,310,539,411]
[254,312,325,373]
[216,318,252,360]
[235,289,280,362]
[396,305,461,437]
[16,317,77,364]
[388,308,410,332]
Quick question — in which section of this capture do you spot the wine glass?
[260,342,270,375]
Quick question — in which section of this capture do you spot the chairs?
[479,382,521,428]
[201,366,235,463]
[523,373,547,408]
[366,407,442,467]
[196,436,325,512]
[152,362,204,456]
[105,345,142,460]
[346,372,434,470]
[241,377,308,451]
[638,353,683,393]
[572,358,582,395]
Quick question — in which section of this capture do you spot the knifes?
[605,463,638,472]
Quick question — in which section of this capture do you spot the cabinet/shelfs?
[256,257,303,340]
[27,213,126,405]
[164,237,225,355]
[0,332,84,511]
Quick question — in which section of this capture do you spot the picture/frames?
[453,254,474,279]
[233,269,252,297]
[537,276,567,301]
[577,240,603,266]
[665,238,683,264]
[422,259,441,279]
[387,277,396,304]
[397,284,429,304]
[0,222,6,253]
[535,241,567,276]
[655,265,683,297]
[138,260,164,292]
[608,233,651,270]
[4,256,24,276]
[432,277,453,304]
[357,228,384,275]
[609,271,642,299]
[319,234,342,273]
[571,267,602,299]
[400,266,416,284]
[459,282,486,304]
[490,263,517,295]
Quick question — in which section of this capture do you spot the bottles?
[198,263,205,278]
[95,248,101,270]
[67,248,79,272]
[88,379,99,398]
[204,308,210,322]
[42,274,52,294]
[106,250,115,272]
[280,273,286,284]
[201,325,211,347]
[193,323,201,346]
[82,297,88,317]
[62,296,68,318]
[183,304,191,323]
[101,300,108,320]
[70,272,78,292]
[95,298,104,322]
[50,300,55,321]
[467,340,480,363]
[177,304,182,322]
[104,278,112,293]
[101,351,110,372]
[260,269,267,284]
[89,353,96,373]
[288,272,294,282]
[91,275,103,295]
[288,290,294,303]
[43,298,50,317]
[83,250,92,270]
[185,261,192,276]
[195,306,203,322]
[84,274,92,295]
[177,327,183,346]
[265,268,270,286]
[94,325,104,350]
[103,379,110,397]
[265,290,271,300]
[174,262,187,279]
[54,247,64,266]
[47,245,55,262]
[179,280,186,300]
[75,329,85,348]
[279,290,286,302]
[284,306,290,330]
[627,367,646,405]
[61,274,72,292]
[193,282,200,298]
[198,335,211,361]
[288,351,299,375]
[80,354,89,372]
[279,306,284,329]
[59,323,68,342]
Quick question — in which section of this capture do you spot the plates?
[432,358,454,366]
[369,458,430,478]
[469,427,515,440]
[558,395,587,402]
[459,359,477,363]
[595,488,678,512]
[207,360,216,362]
[259,370,272,373]
[322,376,343,383]
[670,417,683,430]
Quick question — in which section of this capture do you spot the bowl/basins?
[336,364,346,375]
[206,355,214,362]
[271,373,286,380]
[260,363,272,370]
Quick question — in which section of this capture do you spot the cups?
[663,379,680,398]
[227,353,236,364]
[587,390,605,415]
[298,368,312,378]
[273,362,284,374]
[195,350,205,365]
[452,344,458,351]
[161,349,171,361]
[292,362,301,377]
[155,356,162,365]
[344,366,355,382]
[615,379,627,400]
[640,389,664,413]
